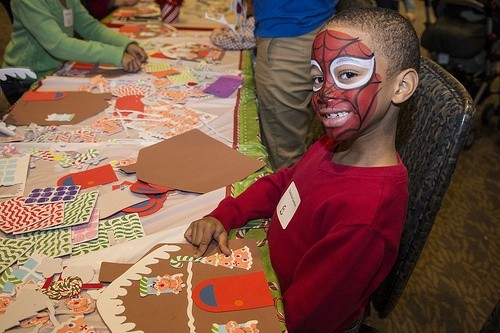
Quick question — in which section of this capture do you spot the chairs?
[358,56,476,333]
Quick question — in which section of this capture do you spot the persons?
[185,6,421,332]
[253,0,338,173]
[2,1,147,106]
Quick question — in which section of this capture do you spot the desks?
[1,0,294,333]
